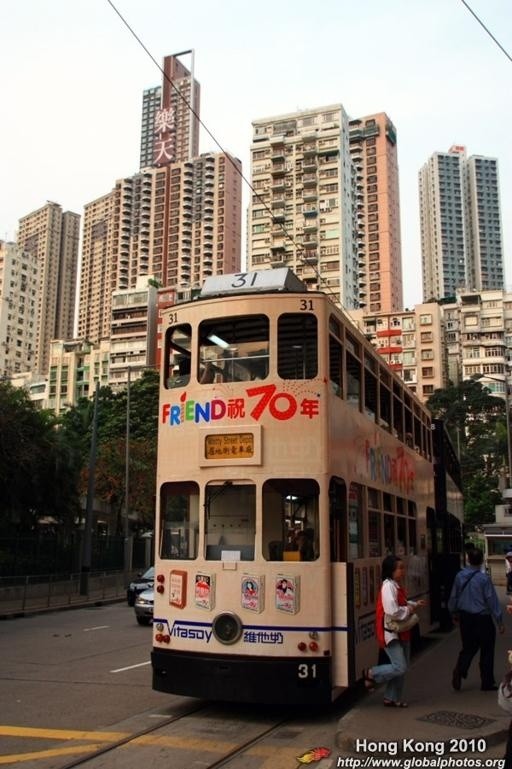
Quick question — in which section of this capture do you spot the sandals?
[383,697,410,708]
[360,664,377,697]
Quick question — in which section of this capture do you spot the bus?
[126,272,467,718]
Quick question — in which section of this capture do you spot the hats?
[504,551,512,559]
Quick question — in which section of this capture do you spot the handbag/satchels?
[384,610,420,632]
[497,678,512,712]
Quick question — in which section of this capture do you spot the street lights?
[469,372,512,490]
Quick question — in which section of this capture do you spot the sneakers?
[449,666,464,693]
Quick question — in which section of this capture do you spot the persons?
[447,543,512,768]
[362,555,428,708]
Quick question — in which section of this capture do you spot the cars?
[133,583,154,628]
[127,563,155,607]
